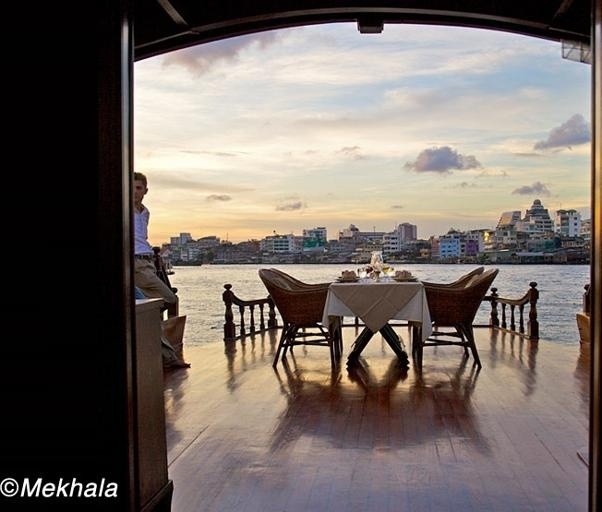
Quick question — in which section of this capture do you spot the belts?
[136,253,154,259]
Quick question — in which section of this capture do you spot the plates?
[334,276,362,282]
[391,276,419,281]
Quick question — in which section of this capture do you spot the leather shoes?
[168,355,192,368]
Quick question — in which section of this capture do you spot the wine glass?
[380,263,390,280]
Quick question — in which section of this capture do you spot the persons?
[133,172,191,371]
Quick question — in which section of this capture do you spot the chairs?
[258,268,343,370]
[407,266,500,377]
